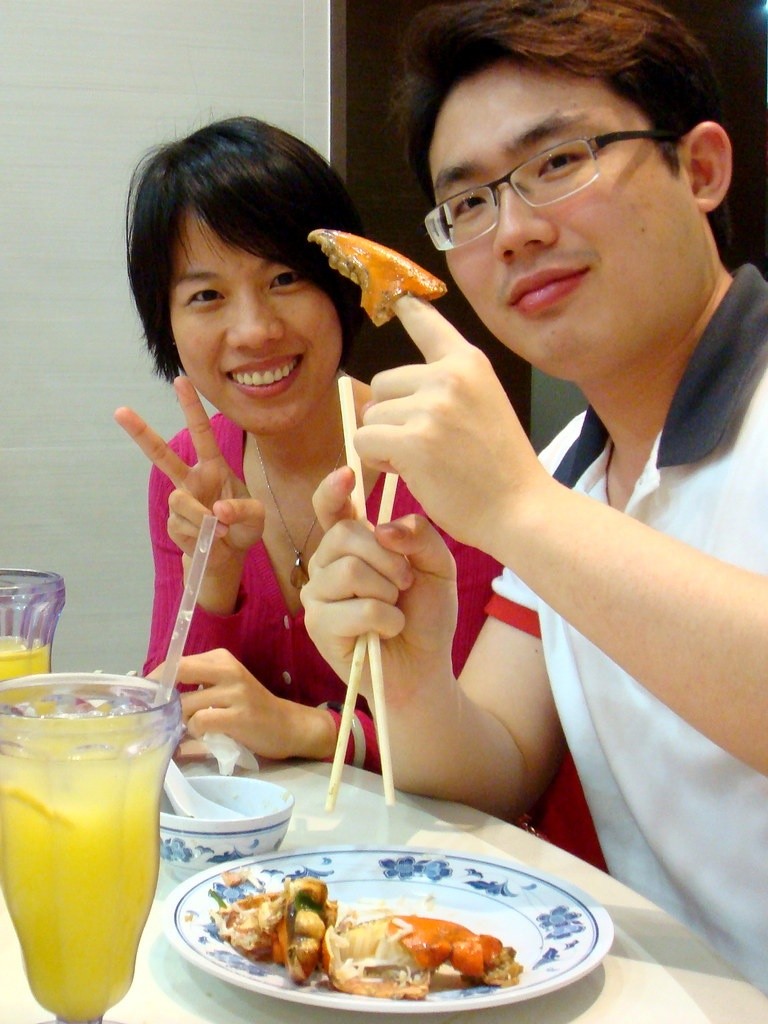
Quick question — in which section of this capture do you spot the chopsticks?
[324,376,400,812]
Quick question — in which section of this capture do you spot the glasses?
[421,131,673,251]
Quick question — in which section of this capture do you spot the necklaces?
[254,436,346,590]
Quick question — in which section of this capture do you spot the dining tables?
[0,743,768,1024]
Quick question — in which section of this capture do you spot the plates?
[163,844,614,1013]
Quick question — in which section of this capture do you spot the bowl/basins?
[159,776,294,881]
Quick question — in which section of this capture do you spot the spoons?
[163,759,248,820]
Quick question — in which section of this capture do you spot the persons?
[296,0,768,1000]
[112,115,609,874]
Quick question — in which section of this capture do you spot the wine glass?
[0,672,182,1024]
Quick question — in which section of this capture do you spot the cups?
[0,568,65,681]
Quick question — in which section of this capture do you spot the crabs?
[208,868,523,1002]
